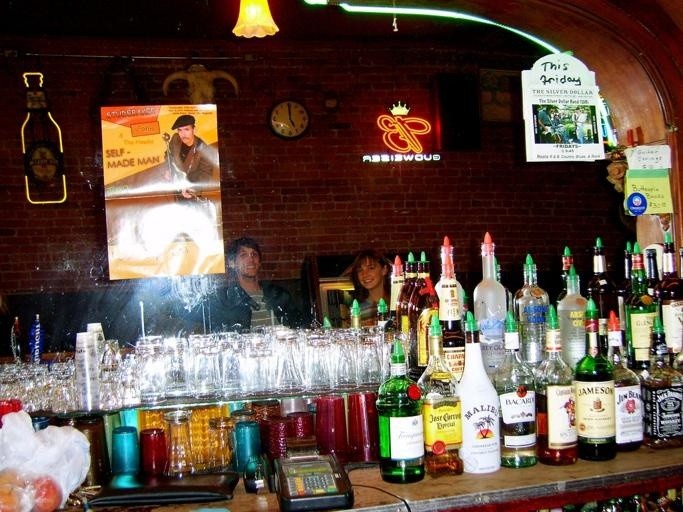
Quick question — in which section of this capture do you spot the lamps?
[232,1,283,43]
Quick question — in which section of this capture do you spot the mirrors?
[0,2,677,384]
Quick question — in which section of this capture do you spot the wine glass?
[0,360,78,413]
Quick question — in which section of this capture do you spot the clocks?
[267,96,313,143]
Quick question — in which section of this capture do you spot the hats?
[172,115,197,132]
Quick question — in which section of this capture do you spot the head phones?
[228,238,246,272]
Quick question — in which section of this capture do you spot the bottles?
[377,336,427,485]
[638,314,683,449]
[418,324,463,481]
[242,455,280,494]
[75,326,383,487]
[5,310,44,364]
[574,297,617,462]
[456,311,503,474]
[320,231,683,383]
[20,72,68,206]
[532,305,579,466]
[605,311,644,451]
[492,311,538,469]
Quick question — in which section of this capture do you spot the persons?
[341,248,391,330]
[166,115,213,203]
[203,237,304,336]
[537,106,588,144]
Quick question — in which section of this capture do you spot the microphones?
[162,133,170,141]
